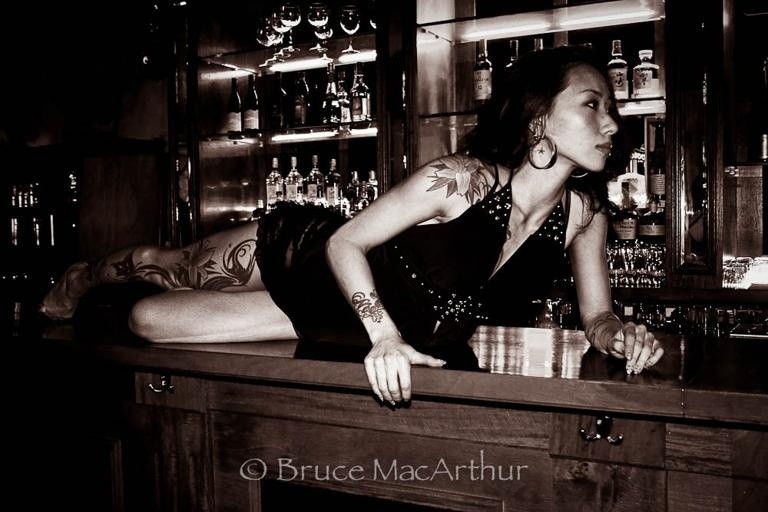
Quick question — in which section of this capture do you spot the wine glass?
[254,2,360,70]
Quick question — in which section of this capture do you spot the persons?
[34,43,666,407]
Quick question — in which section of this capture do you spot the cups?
[722,256,768,294]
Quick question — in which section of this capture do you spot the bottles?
[3,170,78,318]
[261,154,379,219]
[529,298,767,339]
[219,67,370,136]
[606,175,668,285]
[470,32,660,104]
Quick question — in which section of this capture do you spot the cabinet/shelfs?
[166,0,399,259]
[683,0,766,309]
[399,0,678,301]
